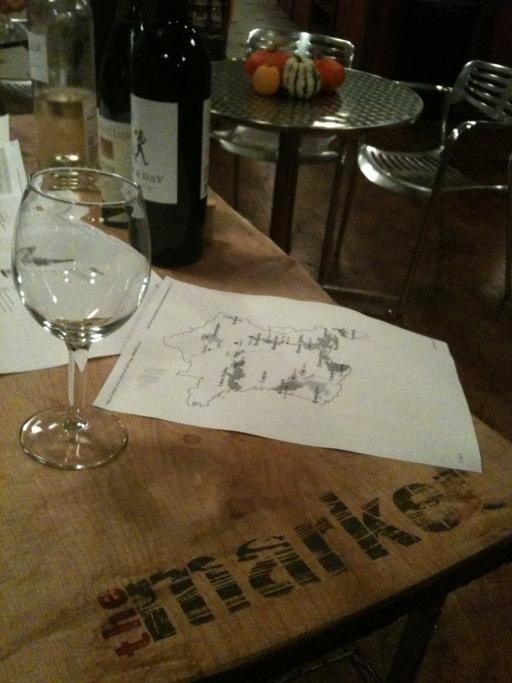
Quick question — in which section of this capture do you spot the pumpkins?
[243,44,345,99]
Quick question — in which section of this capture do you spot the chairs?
[220,25,355,218]
[334,60,509,316]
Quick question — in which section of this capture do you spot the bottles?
[24,0,99,194]
[127,0,212,268]
[94,1,156,228]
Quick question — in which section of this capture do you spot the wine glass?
[10,164,154,472]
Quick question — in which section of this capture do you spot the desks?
[212,59,422,256]
